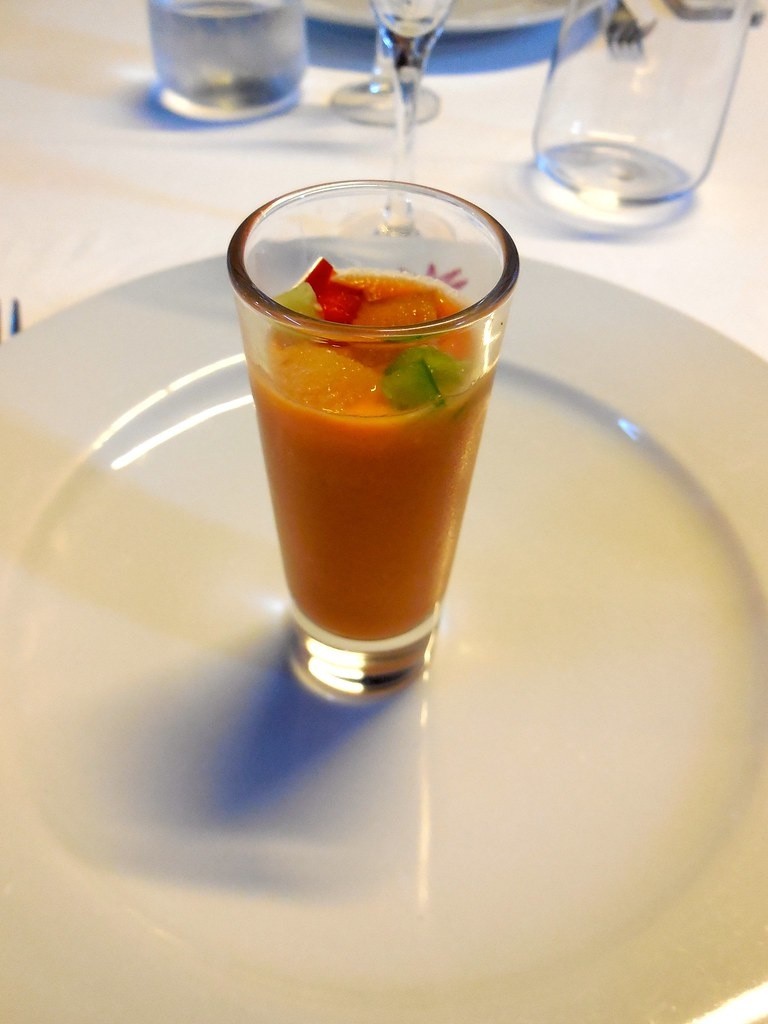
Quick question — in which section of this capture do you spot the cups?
[533,1,753,230]
[228,178,520,700]
[145,0,311,123]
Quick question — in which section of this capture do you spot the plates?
[255,0,598,32]
[0,243,767,1023]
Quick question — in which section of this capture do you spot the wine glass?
[332,0,452,126]
[343,0,463,245]
